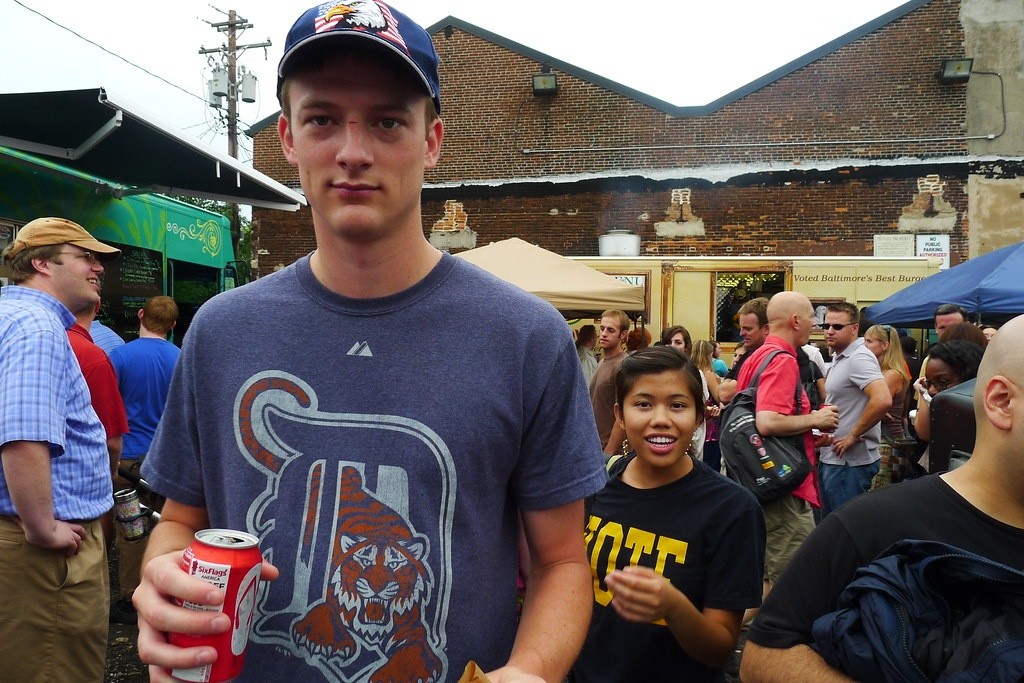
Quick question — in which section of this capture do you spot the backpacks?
[719,350,813,505]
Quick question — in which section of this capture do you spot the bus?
[0,143,250,360]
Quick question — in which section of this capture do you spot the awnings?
[0,84,307,212]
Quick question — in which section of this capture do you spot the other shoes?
[110,594,140,625]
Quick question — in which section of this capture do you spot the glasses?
[821,322,858,331]
[49,249,98,264]
[921,376,964,392]
[883,324,891,344]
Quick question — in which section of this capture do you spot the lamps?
[937,57,974,85]
[532,73,557,95]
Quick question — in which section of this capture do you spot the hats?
[276,0,442,114]
[8,216,123,263]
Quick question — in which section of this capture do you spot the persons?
[65,278,181,622]
[717,290,997,653]
[576,309,748,485]
[131,0,608,683]
[0,217,122,683]
[740,314,1024,683]
[563,345,767,683]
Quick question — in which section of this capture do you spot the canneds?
[165,529,263,683]
[818,404,836,433]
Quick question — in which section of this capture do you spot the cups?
[115,488,145,538]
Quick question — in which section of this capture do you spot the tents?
[451,237,645,349]
[864,241,1024,357]
